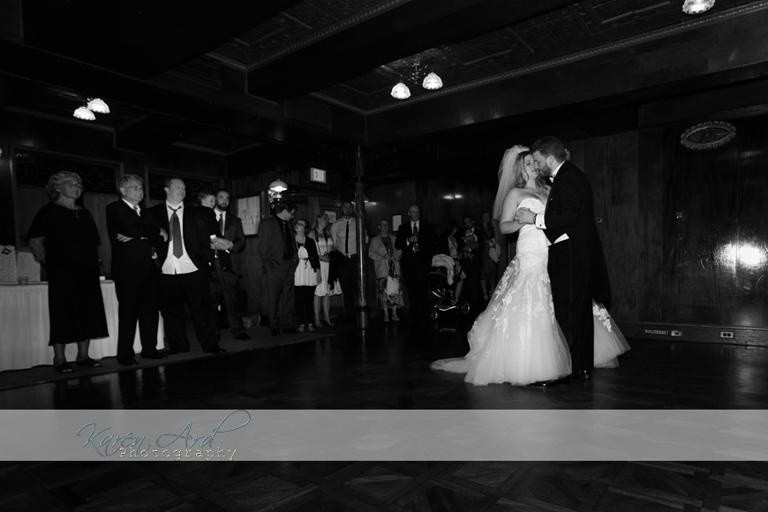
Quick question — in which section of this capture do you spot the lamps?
[71,93,111,125]
[679,0,719,17]
[388,53,445,101]
[269,175,288,206]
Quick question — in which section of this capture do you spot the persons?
[516,136,614,386]
[430,143,634,388]
[25,171,509,375]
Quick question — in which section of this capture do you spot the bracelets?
[532,214,538,225]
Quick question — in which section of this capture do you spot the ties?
[168,205,183,259]
[218,214,224,235]
[412,222,419,252]
[345,221,349,253]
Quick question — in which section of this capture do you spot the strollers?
[427,255,475,320]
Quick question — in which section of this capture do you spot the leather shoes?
[116,357,139,366]
[554,369,591,388]
[54,363,73,373]
[141,353,170,359]
[168,347,190,354]
[78,357,102,368]
[202,344,227,355]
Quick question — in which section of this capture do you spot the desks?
[0,270,169,376]
